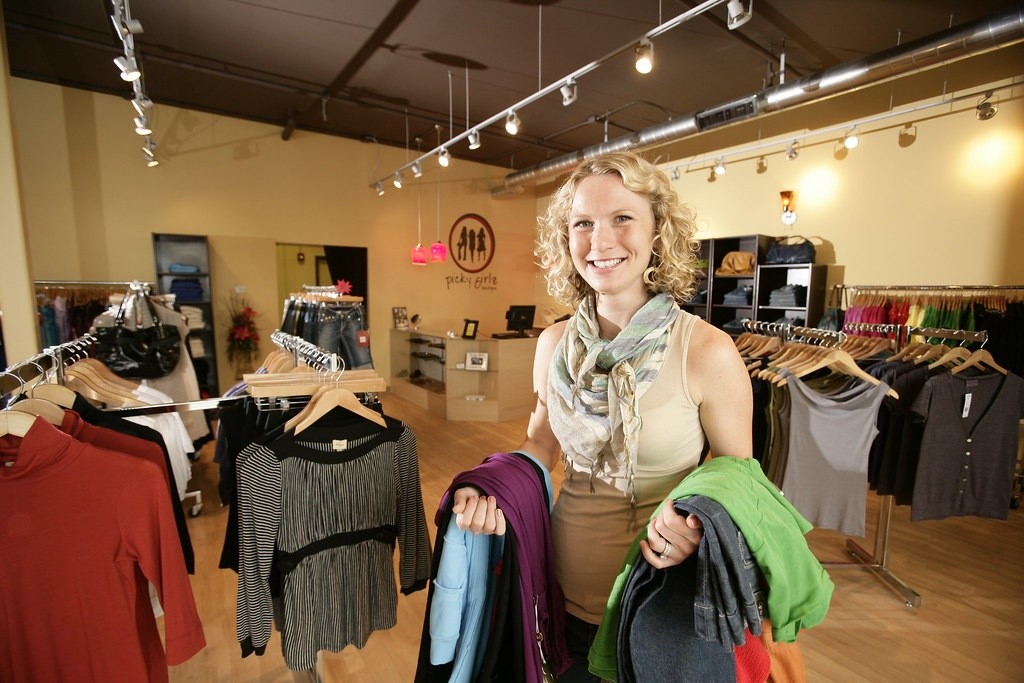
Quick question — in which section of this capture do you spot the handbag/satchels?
[93,284,181,380]
[715,251,756,275]
[818,285,848,331]
[762,235,818,264]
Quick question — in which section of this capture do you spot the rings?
[660,542,671,559]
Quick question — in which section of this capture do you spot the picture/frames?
[466,352,489,372]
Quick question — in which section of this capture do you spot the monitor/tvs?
[507,306,536,337]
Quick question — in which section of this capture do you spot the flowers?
[220,294,263,368]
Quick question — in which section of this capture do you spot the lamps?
[411,162,424,179]
[842,125,861,148]
[394,171,405,189]
[412,138,428,264]
[505,108,521,135]
[468,129,482,150]
[669,166,682,181]
[786,139,799,159]
[376,181,384,196]
[431,124,447,260]
[634,37,654,74]
[439,147,452,168]
[976,87,999,120]
[110,1,159,166]
[560,76,578,106]
[714,156,725,175]
[727,0,752,31]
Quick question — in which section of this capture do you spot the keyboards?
[492,333,518,339]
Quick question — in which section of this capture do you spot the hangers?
[0,283,181,439]
[290,284,363,306]
[734,286,1024,398]
[242,331,387,435]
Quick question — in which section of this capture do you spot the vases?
[237,350,255,379]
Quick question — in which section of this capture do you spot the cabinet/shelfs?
[680,234,827,335]
[389,328,446,416]
[153,233,220,400]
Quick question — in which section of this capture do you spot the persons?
[51,293,77,345]
[86,299,105,329]
[452,152,753,683]
[38,298,57,347]
